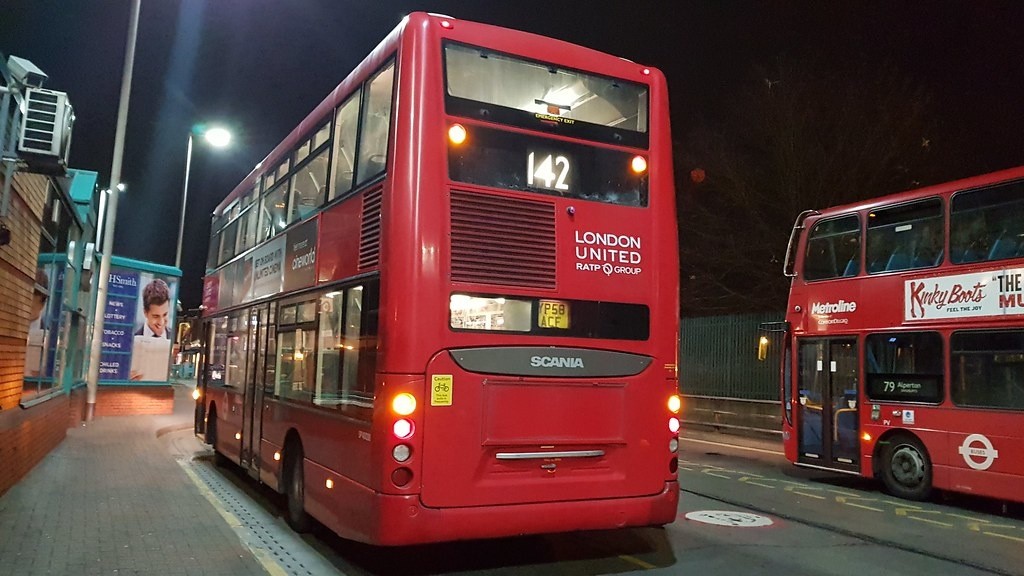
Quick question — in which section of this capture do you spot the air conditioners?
[18,88,76,169]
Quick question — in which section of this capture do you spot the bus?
[759,166,1024,503]
[195,12,680,547]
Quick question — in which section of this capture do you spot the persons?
[24,270,50,377]
[130,278,172,382]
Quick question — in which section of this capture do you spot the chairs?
[268,154,385,239]
[843,230,1024,277]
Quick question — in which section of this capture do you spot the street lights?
[225,330,239,385]
[175,128,224,268]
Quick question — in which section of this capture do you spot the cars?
[282,347,303,361]
